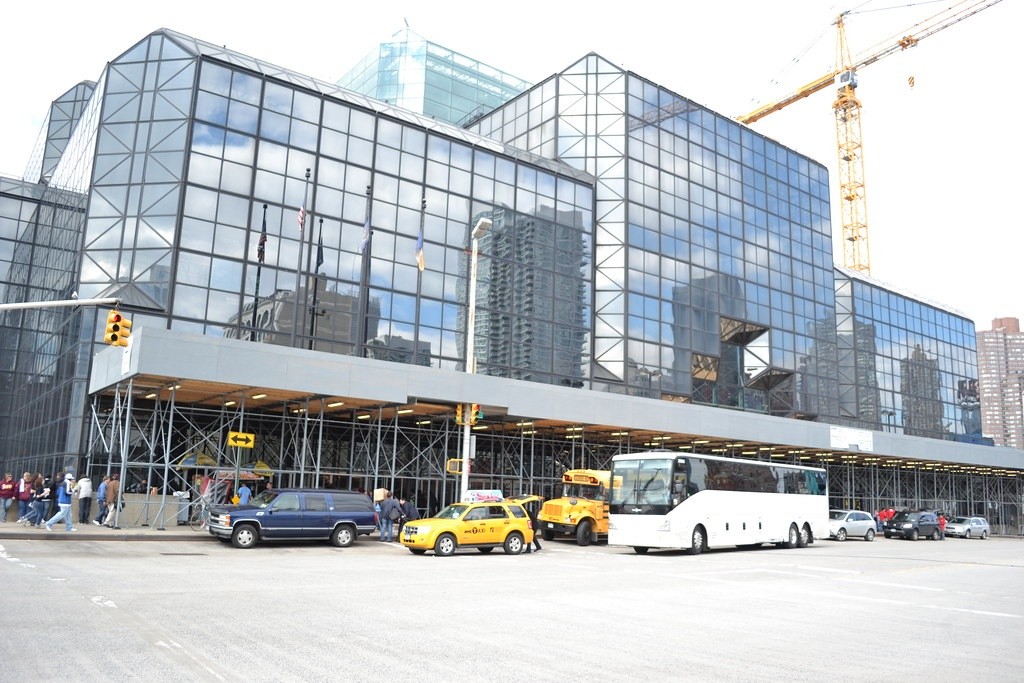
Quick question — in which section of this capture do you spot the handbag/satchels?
[115,494,125,509]
[390,508,400,520]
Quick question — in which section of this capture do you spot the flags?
[297,199,306,230]
[359,199,371,265]
[415,210,424,272]
[257,220,267,264]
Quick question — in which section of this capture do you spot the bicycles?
[189,494,214,532]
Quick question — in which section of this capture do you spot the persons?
[877,505,896,533]
[0,471,124,532]
[265,482,274,504]
[237,482,252,504]
[644,479,664,491]
[595,492,608,501]
[937,515,953,541]
[362,489,439,542]
[522,509,541,553]
[137,479,148,494]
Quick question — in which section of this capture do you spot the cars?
[828,508,877,542]
[399,493,545,557]
[944,515,991,540]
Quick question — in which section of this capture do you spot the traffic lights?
[447,457,460,474]
[113,316,132,347]
[105,311,123,343]
[471,403,480,424]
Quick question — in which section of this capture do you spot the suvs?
[205,487,378,548]
[882,507,943,540]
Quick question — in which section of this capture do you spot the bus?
[536,468,685,547]
[607,447,830,554]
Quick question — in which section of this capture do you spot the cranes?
[728,0,1010,280]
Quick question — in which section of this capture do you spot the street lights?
[457,217,495,503]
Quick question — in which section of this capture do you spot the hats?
[114,474,119,480]
[65,473,74,479]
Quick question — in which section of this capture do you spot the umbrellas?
[175,451,218,491]
[216,460,274,497]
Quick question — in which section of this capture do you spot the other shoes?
[534,546,541,553]
[45,523,52,532]
[522,551,531,554]
[71,528,76,532]
[103,524,113,528]
[93,520,100,526]
